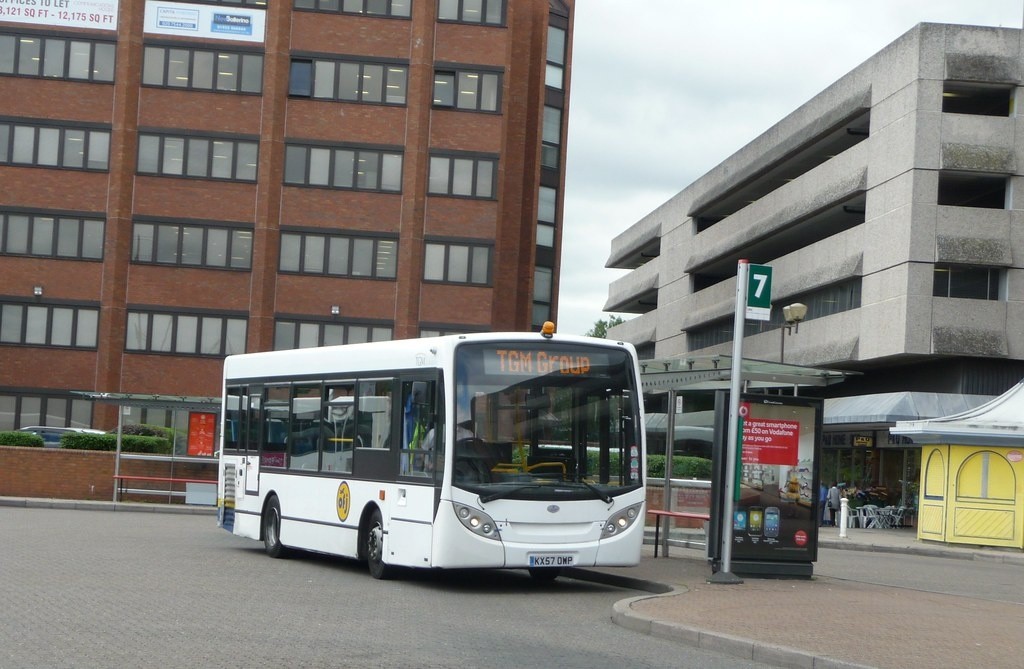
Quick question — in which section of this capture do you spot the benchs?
[646,509,710,559]
[112,473,219,503]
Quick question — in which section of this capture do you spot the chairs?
[847,502,911,530]
[230,418,346,451]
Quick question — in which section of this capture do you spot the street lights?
[779,303,807,364]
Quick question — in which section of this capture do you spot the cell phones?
[734,506,780,538]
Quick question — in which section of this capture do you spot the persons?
[826,481,841,527]
[819,481,827,527]
[404,405,473,473]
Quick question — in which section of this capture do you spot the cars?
[19,424,108,448]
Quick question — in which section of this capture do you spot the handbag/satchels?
[828,502,832,507]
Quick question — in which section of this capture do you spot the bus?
[212,319,648,581]
